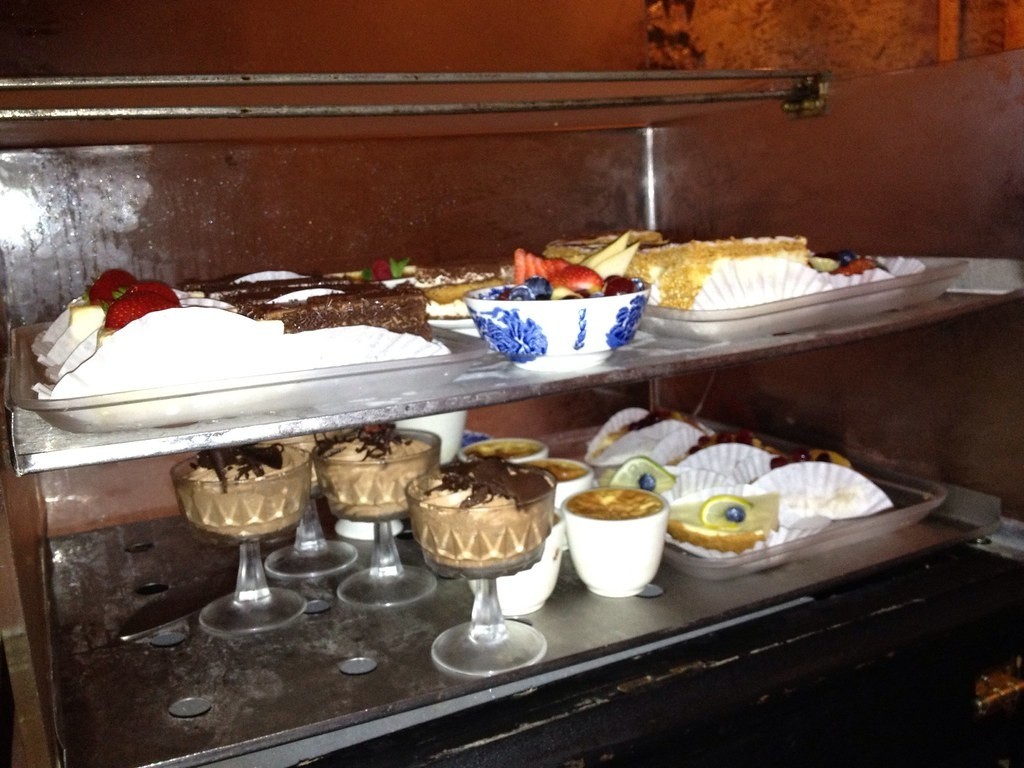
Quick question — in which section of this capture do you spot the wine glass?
[170,427,354,635]
[311,428,437,607]
[405,461,557,676]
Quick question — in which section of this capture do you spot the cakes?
[542,229,811,311]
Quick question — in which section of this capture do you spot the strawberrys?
[513,247,604,293]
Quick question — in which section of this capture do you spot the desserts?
[587,404,894,564]
[169,422,665,579]
[33,256,507,403]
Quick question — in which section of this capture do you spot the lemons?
[607,456,677,496]
[701,495,753,531]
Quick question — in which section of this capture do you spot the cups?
[468,512,566,617]
[525,458,594,508]
[458,437,547,464]
[562,486,670,597]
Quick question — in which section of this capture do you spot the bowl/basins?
[392,410,467,465]
[463,281,651,371]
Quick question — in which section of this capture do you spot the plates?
[917,257,1022,296]
[231,269,480,340]
